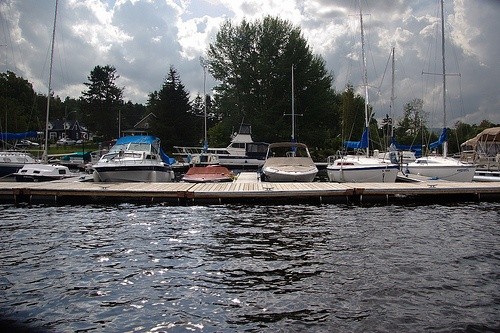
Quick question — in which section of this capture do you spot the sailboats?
[182,68,236,182]
[12,0,81,182]
[258,64,318,182]
[327,1,499,183]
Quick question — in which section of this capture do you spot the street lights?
[63,110,78,140]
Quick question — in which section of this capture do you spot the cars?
[57,138,77,146]
[76,140,88,144]
[108,139,118,146]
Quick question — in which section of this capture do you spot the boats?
[91,112,175,183]
[0,131,44,170]
[172,117,272,168]
